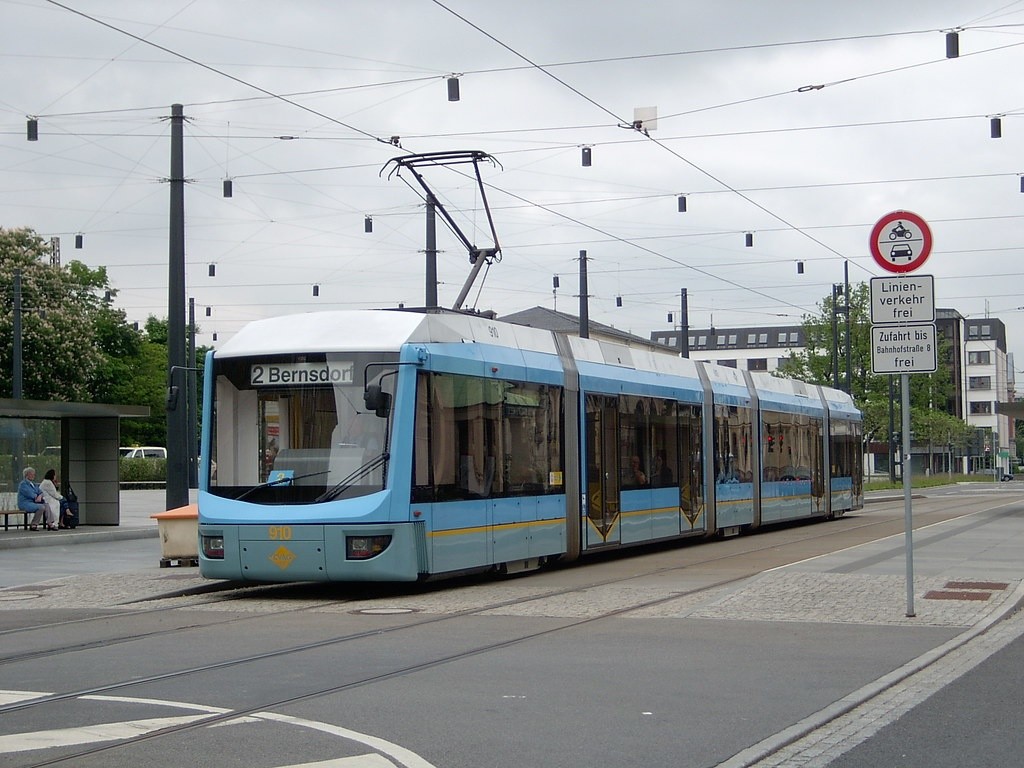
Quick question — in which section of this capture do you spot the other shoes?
[59,524,70,529]
[30,524,40,531]
[48,525,58,531]
[67,512,74,516]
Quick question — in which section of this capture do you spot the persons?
[15,464,64,533]
[38,466,75,533]
[624,455,674,486]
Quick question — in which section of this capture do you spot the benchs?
[0,510,47,532]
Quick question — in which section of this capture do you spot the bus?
[165,149,864,585]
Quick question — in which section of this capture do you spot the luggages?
[63,501,79,529]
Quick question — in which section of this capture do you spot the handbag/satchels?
[66,480,77,501]
[34,493,44,503]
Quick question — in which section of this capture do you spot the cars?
[1001,474,1013,483]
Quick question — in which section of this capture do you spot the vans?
[119,446,167,461]
